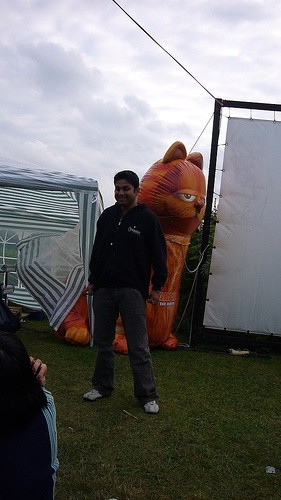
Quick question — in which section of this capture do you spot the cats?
[55,141,206,355]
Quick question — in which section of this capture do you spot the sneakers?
[83,389,103,401]
[144,400,159,414]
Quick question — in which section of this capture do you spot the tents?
[0,156,105,348]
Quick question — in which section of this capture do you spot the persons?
[0,331,60,500]
[82,171,168,413]
